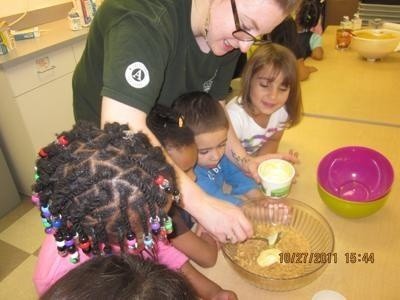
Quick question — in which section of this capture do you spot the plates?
[380,23,400,53]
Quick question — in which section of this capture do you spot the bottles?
[336,13,362,49]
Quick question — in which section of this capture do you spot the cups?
[257,158,296,200]
[67,17,82,31]
[311,289,346,300]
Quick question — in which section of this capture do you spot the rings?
[230,233,236,241]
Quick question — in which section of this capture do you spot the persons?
[72,0,297,246]
[30,119,238,300]
[41,255,204,300]
[247,17,318,82]
[145,103,220,269]
[294,0,323,60]
[170,90,294,231]
[223,42,305,157]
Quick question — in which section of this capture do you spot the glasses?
[230,0,272,45]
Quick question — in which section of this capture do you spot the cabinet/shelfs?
[0,39,107,220]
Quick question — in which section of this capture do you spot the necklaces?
[204,2,213,41]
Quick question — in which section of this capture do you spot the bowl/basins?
[218,196,336,292]
[350,29,400,61]
[316,146,395,220]
